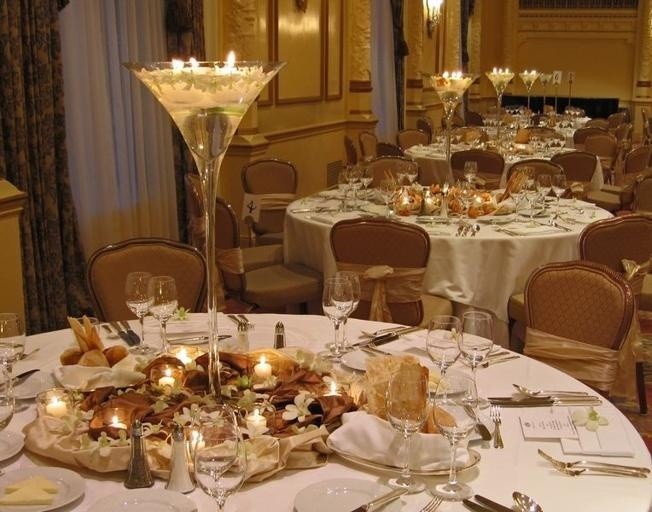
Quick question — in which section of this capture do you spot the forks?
[538,449,652,482]
[515,386,604,406]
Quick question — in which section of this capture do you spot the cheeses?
[0,476,58,505]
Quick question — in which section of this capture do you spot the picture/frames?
[257,0,344,108]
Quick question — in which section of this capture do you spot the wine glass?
[487,64,574,130]
[1,272,546,512]
[438,107,586,154]
[120,55,288,404]
[424,67,481,154]
[336,158,558,229]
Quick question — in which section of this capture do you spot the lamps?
[426,0,439,39]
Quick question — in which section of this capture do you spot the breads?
[385,362,457,433]
[60,315,128,368]
